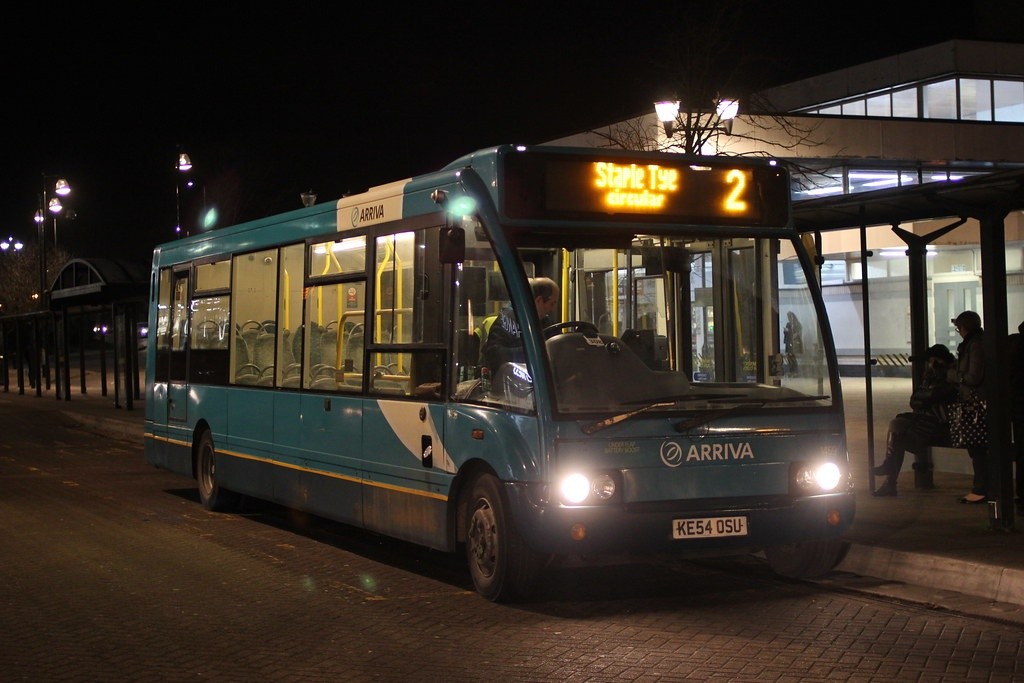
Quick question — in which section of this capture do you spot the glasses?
[953,324,965,332]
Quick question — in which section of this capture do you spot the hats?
[928,344,949,358]
[952,311,981,332]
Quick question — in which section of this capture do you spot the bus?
[143,143,854,600]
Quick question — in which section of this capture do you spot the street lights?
[649,87,742,392]
[171,146,192,241]
[31,170,73,397]
[0,235,23,312]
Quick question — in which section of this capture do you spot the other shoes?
[956,496,987,505]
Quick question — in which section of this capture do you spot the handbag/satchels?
[944,381,992,449]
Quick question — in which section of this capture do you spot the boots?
[870,432,906,478]
[872,473,897,496]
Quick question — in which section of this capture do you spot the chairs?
[172,320,415,396]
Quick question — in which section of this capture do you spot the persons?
[872,311,1024,504]
[481,278,563,379]
[783,311,804,377]
